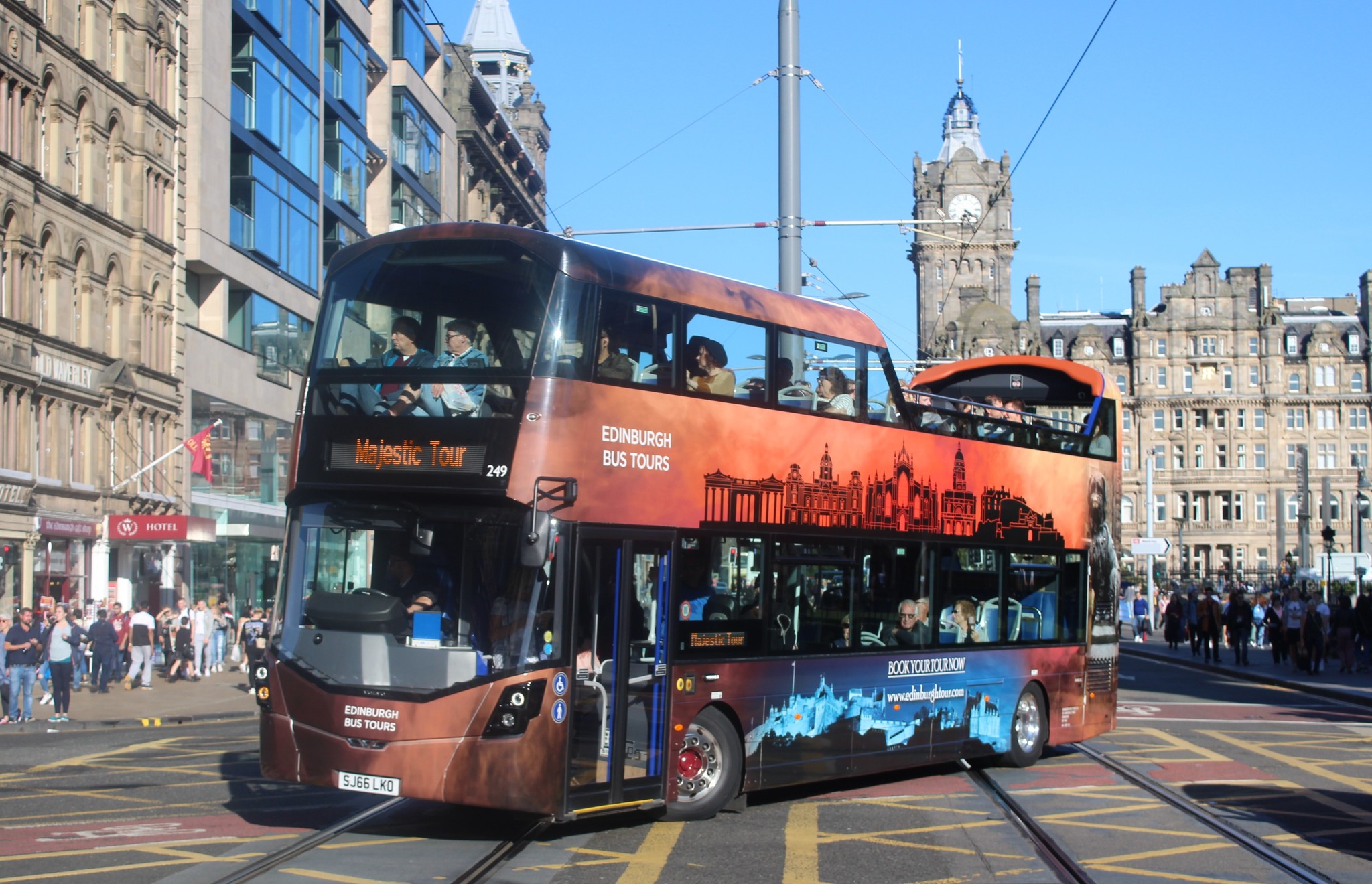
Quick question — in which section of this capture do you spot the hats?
[444,318,477,342]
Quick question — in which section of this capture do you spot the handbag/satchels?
[440,380,477,419]
[231,643,239,661]
[256,636,266,650]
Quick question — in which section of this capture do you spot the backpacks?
[1253,601,1265,621]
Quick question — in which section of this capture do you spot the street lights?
[1320,524,1336,608]
[1356,459,1372,552]
[1172,518,1187,580]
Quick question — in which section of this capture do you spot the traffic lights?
[727,547,738,564]
[13,544,21,565]
[2,544,13,564]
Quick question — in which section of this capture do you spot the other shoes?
[17,709,23,722]
[1134,635,1144,643]
[47,716,61,723]
[71,663,223,694]
[49,698,55,705]
[25,716,35,723]
[1191,645,1372,677]
[0,715,10,725]
[8,718,17,724]
[39,693,52,705]
[61,715,69,722]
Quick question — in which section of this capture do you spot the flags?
[182,424,217,486]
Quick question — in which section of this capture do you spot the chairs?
[542,355,1075,453]
[934,576,1070,646]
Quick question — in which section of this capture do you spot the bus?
[256,219,1124,831]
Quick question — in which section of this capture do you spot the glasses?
[899,613,917,618]
[951,610,962,614]
[445,333,463,340]
[0,620,9,623]
[816,377,830,383]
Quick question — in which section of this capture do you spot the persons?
[948,601,990,643]
[1115,558,1371,679]
[731,570,846,641]
[905,591,942,629]
[374,319,489,418]
[831,613,863,648]
[681,335,734,399]
[879,600,929,650]
[575,323,632,379]
[374,549,446,646]
[341,316,435,417]
[647,549,718,651]
[746,356,1113,458]
[0,587,280,723]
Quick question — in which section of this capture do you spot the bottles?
[22,639,33,653]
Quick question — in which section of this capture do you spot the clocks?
[949,192,981,226]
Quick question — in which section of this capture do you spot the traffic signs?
[1131,537,1171,554]
